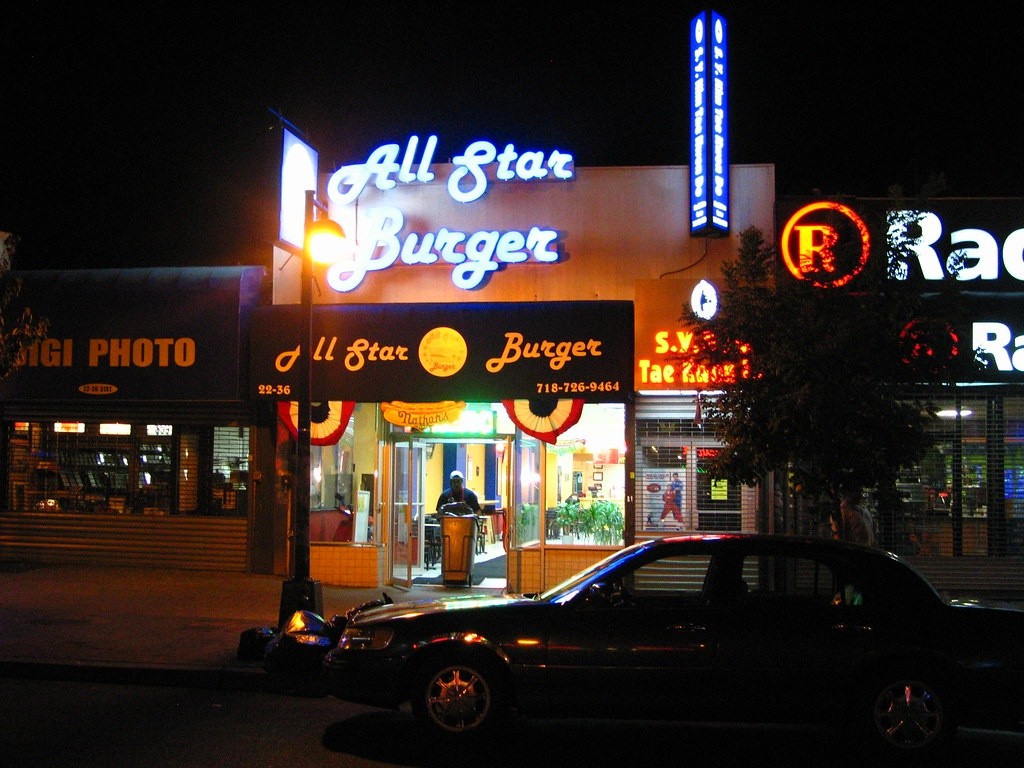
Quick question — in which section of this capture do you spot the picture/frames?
[593,472,603,482]
[593,465,603,469]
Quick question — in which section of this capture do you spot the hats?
[450,470,464,479]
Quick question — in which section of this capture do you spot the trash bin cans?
[439,513,480,588]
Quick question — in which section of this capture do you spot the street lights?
[278,188,346,631]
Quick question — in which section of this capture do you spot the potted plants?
[549,501,625,546]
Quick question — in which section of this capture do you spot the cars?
[322,532,1023,760]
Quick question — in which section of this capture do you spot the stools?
[424,513,494,573]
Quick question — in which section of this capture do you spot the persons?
[648,511,655,523]
[434,470,482,523]
[660,472,685,527]
